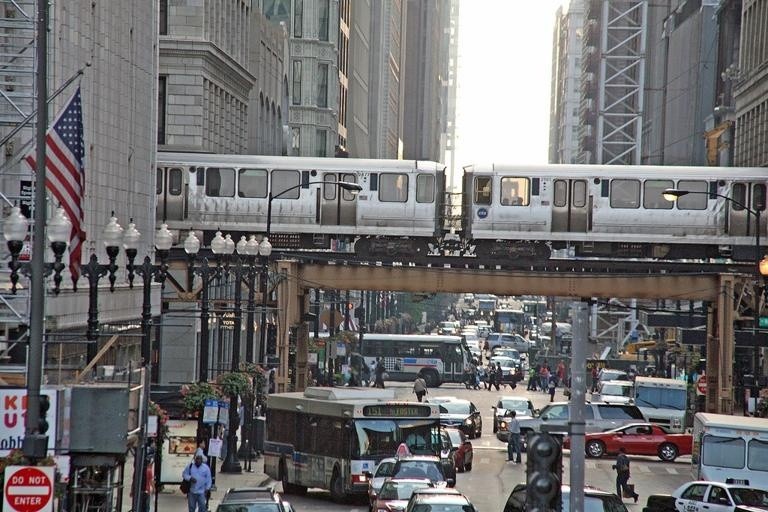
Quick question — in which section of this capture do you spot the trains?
[156,148,767,260]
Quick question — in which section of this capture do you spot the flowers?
[149,361,267,442]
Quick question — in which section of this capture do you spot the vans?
[634,376,687,433]
[518,403,647,450]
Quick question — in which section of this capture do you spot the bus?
[263,386,442,499]
[690,412,768,487]
[309,333,475,387]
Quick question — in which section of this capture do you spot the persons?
[502,197,523,206]
[182,447,213,511]
[559,406,568,417]
[412,373,429,402]
[419,295,661,402]
[506,411,522,463]
[362,356,387,388]
[611,446,639,503]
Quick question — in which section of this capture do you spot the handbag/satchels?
[180,479,191,494]
[422,390,426,395]
[623,484,634,497]
[548,383,555,389]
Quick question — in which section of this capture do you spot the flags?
[23,87,86,284]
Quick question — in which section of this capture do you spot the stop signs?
[1,466,55,512]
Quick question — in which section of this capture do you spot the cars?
[563,422,693,462]
[591,367,635,405]
[216,487,293,512]
[643,477,768,512]
[368,396,482,512]
[438,297,570,387]
[493,397,532,432]
[504,482,628,512]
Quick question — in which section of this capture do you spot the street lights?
[123,220,172,511]
[4,207,71,294]
[663,188,768,414]
[77,212,122,377]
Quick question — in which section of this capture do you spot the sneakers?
[506,459,521,462]
[634,494,638,502]
